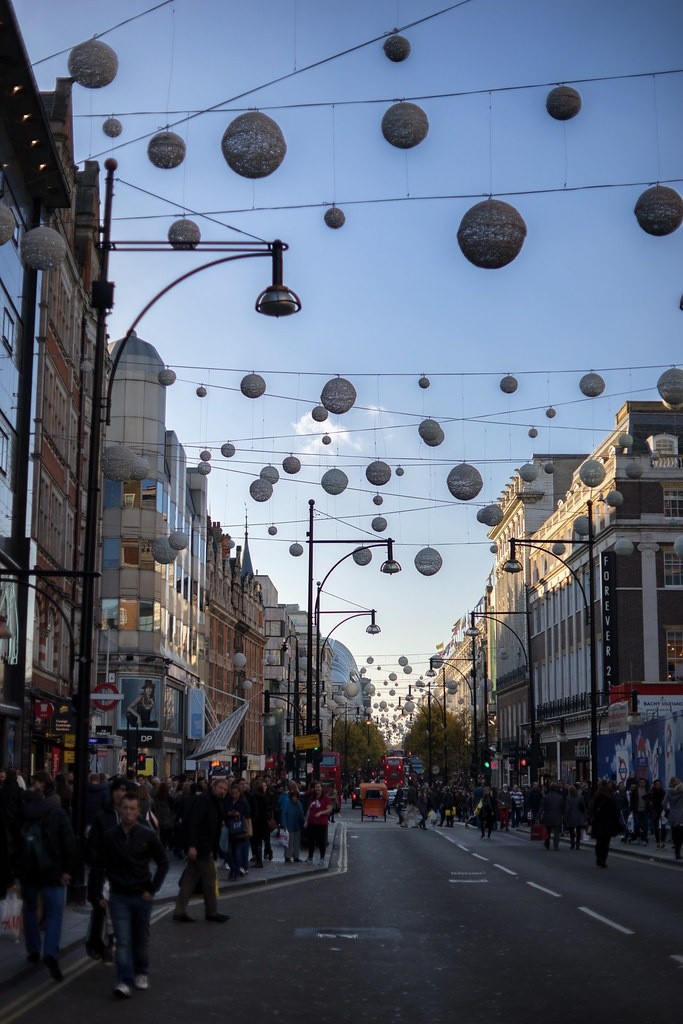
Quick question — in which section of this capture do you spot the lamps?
[145,656,155,662]
[126,654,134,660]
[164,658,173,665]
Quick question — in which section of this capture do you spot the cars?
[350,788,417,808]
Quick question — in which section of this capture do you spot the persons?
[0,767,338,997]
[386,776,683,867]
[341,768,381,804]
[126,679,159,728]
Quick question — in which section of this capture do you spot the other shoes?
[205,912,230,924]
[657,844,667,850]
[227,865,250,882]
[134,974,149,990]
[26,952,40,965]
[319,858,325,866]
[294,858,302,862]
[285,858,292,863]
[173,913,196,923]
[112,982,131,999]
[42,952,64,982]
[304,856,313,864]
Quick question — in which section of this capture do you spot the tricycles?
[359,782,388,822]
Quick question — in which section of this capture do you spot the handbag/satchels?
[217,825,232,858]
[527,809,534,820]
[145,809,159,835]
[269,827,290,848]
[627,813,635,833]
[474,799,483,816]
[589,818,598,838]
[229,813,252,840]
[445,806,456,817]
[0,890,24,945]
[86,900,117,968]
[658,809,671,831]
[531,816,549,841]
[499,804,510,826]
[427,809,442,825]
[467,815,481,828]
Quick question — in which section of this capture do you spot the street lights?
[65,159,303,906]
[466,610,540,783]
[313,609,381,780]
[395,657,480,783]
[306,537,401,786]
[281,633,299,774]
[502,538,598,790]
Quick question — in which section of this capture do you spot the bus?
[406,755,425,785]
[319,752,343,812]
[381,749,406,787]
